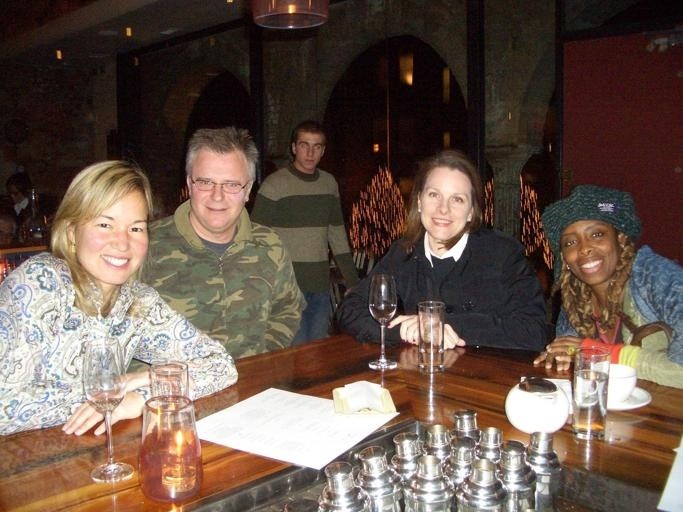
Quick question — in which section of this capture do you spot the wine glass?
[80,333,135,485]
[366,271,399,371]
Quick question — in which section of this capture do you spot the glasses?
[190,175,247,193]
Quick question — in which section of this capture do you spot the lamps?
[252,0,331,30]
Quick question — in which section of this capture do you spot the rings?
[566,346,575,355]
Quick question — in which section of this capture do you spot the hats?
[541,184,641,281]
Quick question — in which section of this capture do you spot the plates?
[601,383,652,415]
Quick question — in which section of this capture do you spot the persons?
[332,147,548,352]
[127,126,310,361]
[0,156,238,438]
[0,172,53,284]
[249,117,362,345]
[531,184,682,381]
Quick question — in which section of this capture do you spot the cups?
[414,297,447,373]
[565,343,610,445]
[140,397,205,505]
[147,358,193,399]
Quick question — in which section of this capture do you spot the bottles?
[26,186,40,222]
[315,407,565,512]
[0,254,33,285]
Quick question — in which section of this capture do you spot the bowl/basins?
[593,359,639,403]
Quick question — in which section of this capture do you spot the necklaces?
[602,306,608,320]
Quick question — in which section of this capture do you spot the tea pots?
[505,375,570,435]
